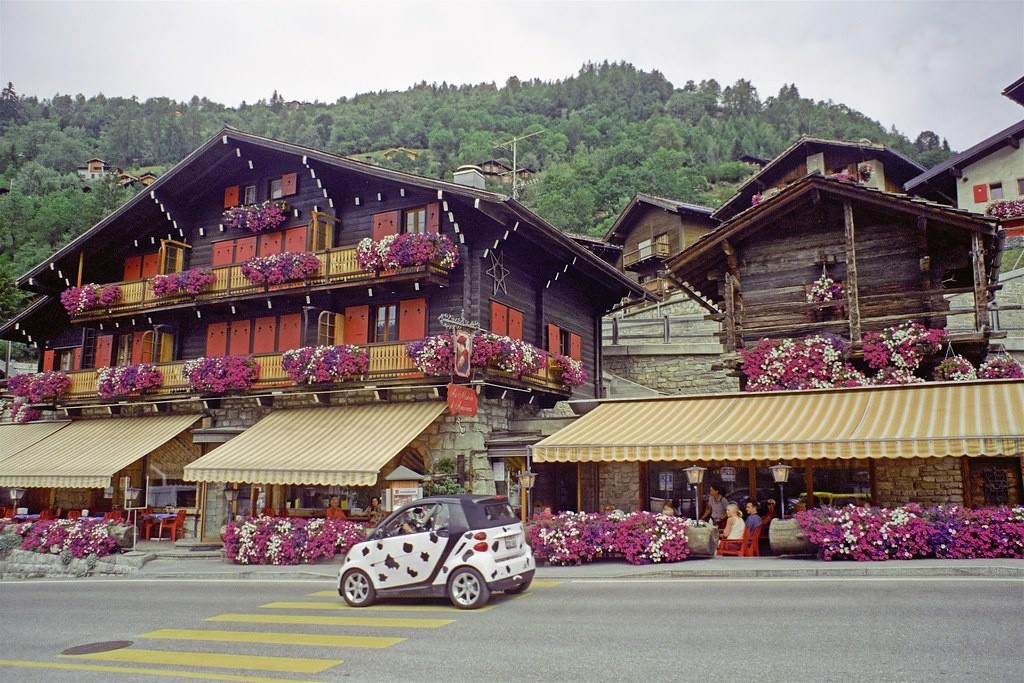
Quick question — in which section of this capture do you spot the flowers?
[794,503,1024,561]
[282,344,368,385]
[529,507,688,565]
[219,515,366,566]
[740,268,1024,393]
[240,252,320,282]
[61,282,120,314]
[355,231,460,273]
[151,267,217,294]
[403,333,587,391]
[94,363,164,400]
[0,371,71,423]
[181,354,260,394]
[835,164,873,184]
[14,520,115,558]
[984,199,1024,222]
[221,202,286,235]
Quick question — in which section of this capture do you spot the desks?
[144,513,177,541]
[77,516,104,522]
[13,514,41,519]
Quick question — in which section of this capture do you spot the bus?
[797,492,871,510]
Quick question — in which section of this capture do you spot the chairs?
[718,524,768,556]
[0,504,187,542]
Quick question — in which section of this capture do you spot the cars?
[727,487,799,514]
[338,493,537,610]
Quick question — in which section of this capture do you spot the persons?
[760,498,776,537]
[662,502,676,517]
[701,485,728,529]
[326,497,346,520]
[744,500,762,546]
[362,497,382,516]
[402,508,449,533]
[713,503,745,556]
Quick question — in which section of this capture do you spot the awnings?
[182,400,448,486]
[0,411,205,488]
[531,380,1024,463]
[0,414,72,488]
